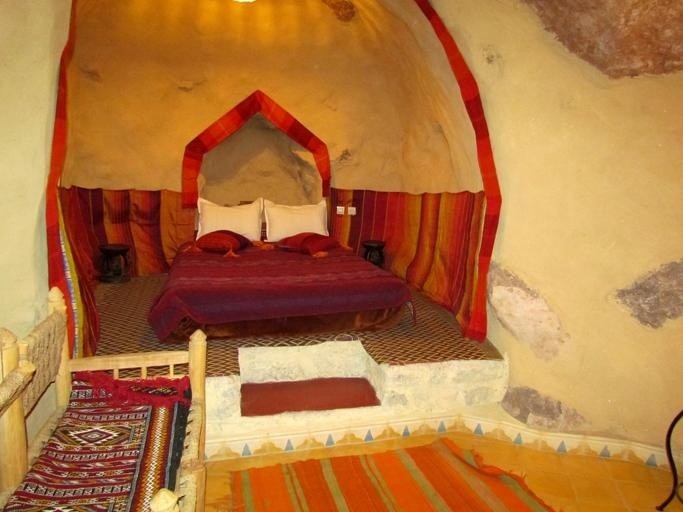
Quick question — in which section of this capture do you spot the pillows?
[196,229,255,252]
[197,201,264,247]
[265,196,330,241]
[274,232,344,255]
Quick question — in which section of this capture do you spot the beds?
[147,229,415,345]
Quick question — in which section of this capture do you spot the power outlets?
[348,207,357,216]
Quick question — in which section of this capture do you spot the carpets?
[91,263,509,389]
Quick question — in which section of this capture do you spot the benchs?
[1,286,208,512]
